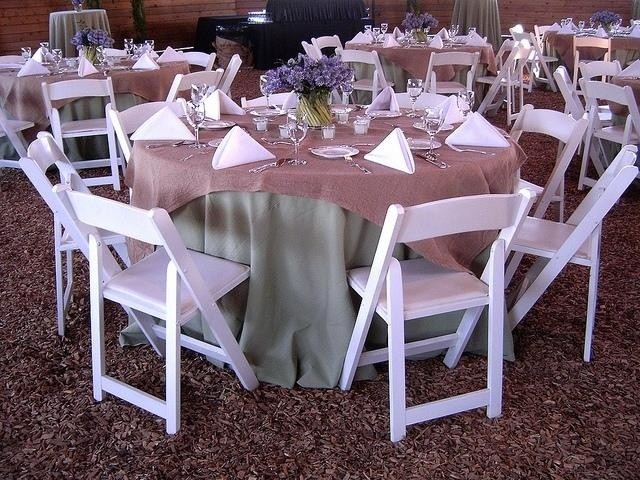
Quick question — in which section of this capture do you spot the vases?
[74,4,82,12]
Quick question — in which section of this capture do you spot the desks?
[195,15,374,70]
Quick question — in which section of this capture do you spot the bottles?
[247,10,273,24]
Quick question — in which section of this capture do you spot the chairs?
[19,130,135,339]
[494,141,638,362]
[338,188,538,441]
[3,24,638,192]
[502,102,589,223]
[46,183,261,436]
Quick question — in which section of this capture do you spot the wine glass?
[363,23,475,45]
[458,90,475,123]
[560,16,624,36]
[21,38,156,75]
[184,70,455,175]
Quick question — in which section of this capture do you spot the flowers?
[400,13,438,41]
[588,10,622,34]
[71,0,85,12]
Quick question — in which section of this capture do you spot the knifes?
[147,140,205,148]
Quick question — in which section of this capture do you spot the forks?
[446,141,495,158]
